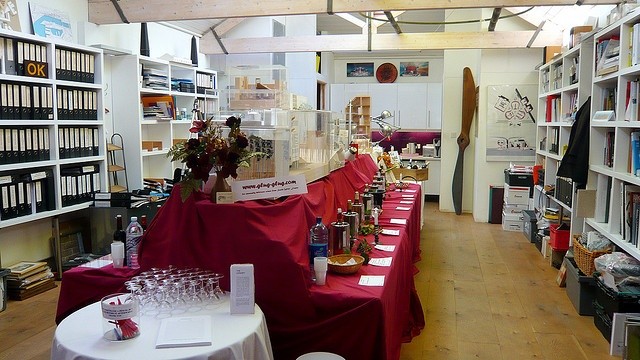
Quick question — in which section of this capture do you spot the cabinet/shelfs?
[583,9,640,262]
[427,82,443,130]
[399,160,441,196]
[369,83,427,129]
[0,29,109,228]
[106,52,219,191]
[329,83,369,118]
[533,39,593,248]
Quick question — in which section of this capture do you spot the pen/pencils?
[108,297,140,341]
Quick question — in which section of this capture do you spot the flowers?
[166,99,219,179]
[209,116,275,179]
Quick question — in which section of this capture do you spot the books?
[627,131,640,176]
[142,95,176,120]
[197,99,217,120]
[553,65,562,90]
[100,178,174,209]
[549,128,558,155]
[625,80,640,121]
[569,57,579,85]
[619,182,640,250]
[0,128,49,163]
[555,177,572,209]
[545,95,561,122]
[628,22,640,67]
[0,36,47,78]
[58,127,99,159]
[197,73,216,94]
[595,35,620,77]
[0,169,56,221]
[534,158,546,188]
[576,173,612,223]
[171,77,196,93]
[58,88,97,120]
[603,131,614,169]
[56,48,95,84]
[5,260,58,301]
[601,85,618,120]
[542,72,550,93]
[1,84,54,119]
[570,94,577,122]
[540,137,546,151]
[139,63,169,90]
[62,163,100,207]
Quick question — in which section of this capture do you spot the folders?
[58,126,99,158]
[92,201,131,209]
[93,192,131,201]
[554,171,573,209]
[0,35,48,79]
[0,81,53,119]
[55,48,96,84]
[0,169,55,221]
[1,126,49,164]
[550,128,559,156]
[197,97,218,120]
[56,86,98,120]
[60,164,100,206]
[171,77,195,93]
[198,72,216,94]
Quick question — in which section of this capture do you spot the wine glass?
[125,264,227,319]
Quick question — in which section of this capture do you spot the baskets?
[572,232,612,278]
[326,254,364,275]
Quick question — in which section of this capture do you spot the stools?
[296,352,345,360]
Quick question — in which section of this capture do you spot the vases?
[211,179,232,203]
[193,179,204,193]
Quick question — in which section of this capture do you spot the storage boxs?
[502,203,529,215]
[534,231,542,253]
[502,183,530,205]
[564,256,595,316]
[521,209,537,243]
[549,224,569,250]
[548,246,565,267]
[593,272,640,345]
[504,169,533,187]
[502,211,523,231]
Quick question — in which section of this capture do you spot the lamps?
[370,110,402,149]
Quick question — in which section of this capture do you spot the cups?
[314,257,328,287]
[111,242,125,269]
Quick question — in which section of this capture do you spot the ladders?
[104,133,129,193]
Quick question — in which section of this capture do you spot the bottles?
[344,200,359,242]
[360,184,373,217]
[308,216,329,281]
[113,215,127,268]
[176,107,180,120]
[352,191,364,224]
[329,208,351,255]
[368,171,385,207]
[123,217,144,269]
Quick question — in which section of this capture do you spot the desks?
[51,291,274,360]
[55,154,425,360]
[55,181,257,325]
